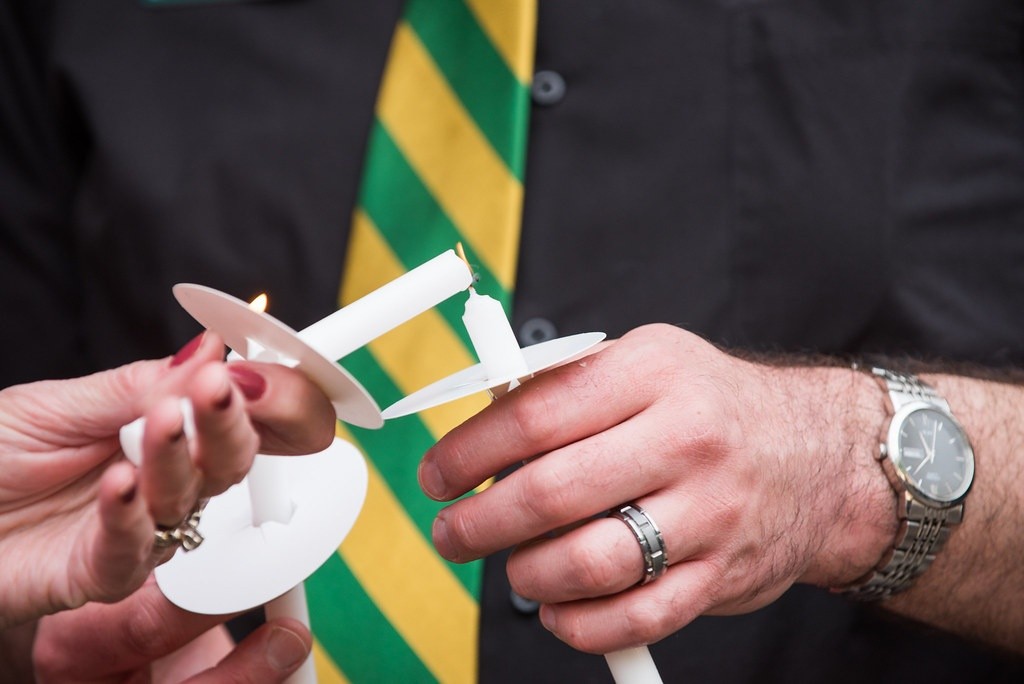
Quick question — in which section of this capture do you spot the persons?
[1,0,1024,684]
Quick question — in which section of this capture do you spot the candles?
[116,249,474,465]
[456,241,665,684]
[226,292,317,684]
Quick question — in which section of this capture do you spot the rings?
[154,500,206,551]
[611,506,667,588]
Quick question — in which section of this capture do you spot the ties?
[261,3,541,683]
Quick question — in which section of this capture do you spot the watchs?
[837,362,975,608]
